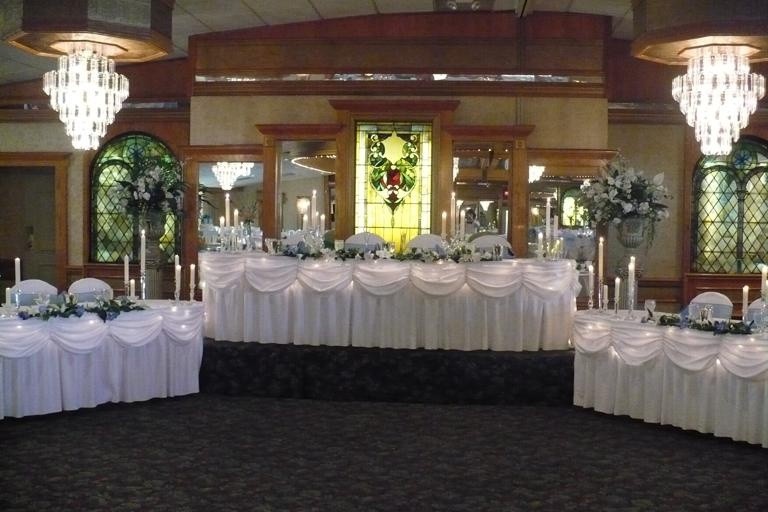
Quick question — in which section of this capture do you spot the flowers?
[91,147,219,219]
[563,149,674,256]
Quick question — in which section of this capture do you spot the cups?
[334,240,344,250]
[6,288,20,309]
[493,242,501,261]
[265,238,278,255]
[697,304,713,322]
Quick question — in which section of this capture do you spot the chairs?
[10,279,58,306]
[689,292,734,319]
[743,298,768,328]
[68,277,114,304]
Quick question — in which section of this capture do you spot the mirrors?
[526,150,612,256]
[452,138,515,247]
[198,161,263,250]
[270,135,342,240]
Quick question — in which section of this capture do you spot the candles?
[175,254,198,300]
[460,211,465,241]
[538,197,559,250]
[589,236,635,303]
[761,266,768,298]
[742,285,750,312]
[303,190,326,231]
[15,257,20,286]
[441,210,446,235]
[6,287,11,308]
[124,229,150,300]
[220,193,238,239]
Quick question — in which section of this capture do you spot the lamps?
[213,160,254,190]
[0,0,190,151]
[629,1,768,156]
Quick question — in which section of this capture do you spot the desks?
[0,300,204,418]
[197,251,581,352]
[573,309,767,450]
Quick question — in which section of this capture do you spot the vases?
[614,217,649,250]
[137,209,167,241]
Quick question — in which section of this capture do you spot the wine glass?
[388,241,395,255]
[644,300,656,312]
